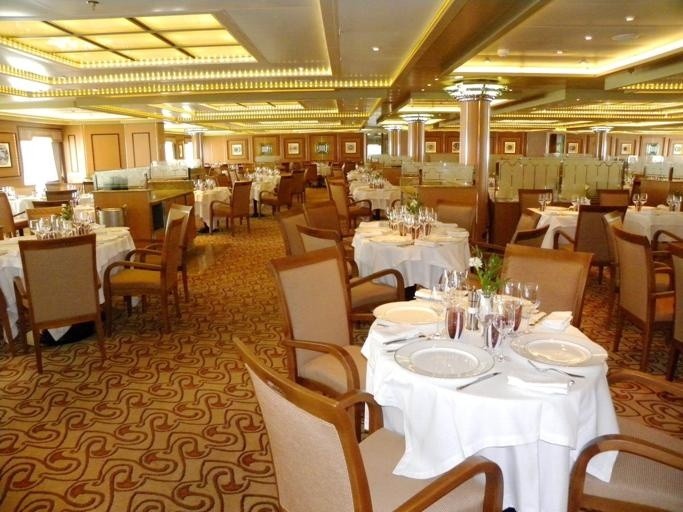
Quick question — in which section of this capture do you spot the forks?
[525,358,587,380]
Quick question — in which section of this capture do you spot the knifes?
[455,372,501,391]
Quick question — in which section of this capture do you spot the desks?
[357,286,625,512]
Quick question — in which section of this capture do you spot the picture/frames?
[0,132,21,178]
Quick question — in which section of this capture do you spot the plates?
[369,234,407,246]
[372,299,441,326]
[508,330,609,369]
[393,338,494,380]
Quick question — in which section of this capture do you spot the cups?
[0,185,16,197]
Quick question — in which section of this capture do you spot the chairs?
[4,163,681,380]
[566,412,682,511]
[227,334,511,511]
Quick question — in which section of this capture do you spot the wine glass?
[535,191,586,213]
[428,266,538,362]
[384,203,440,242]
[195,158,280,190]
[29,213,91,238]
[630,193,682,213]
[353,165,388,189]
